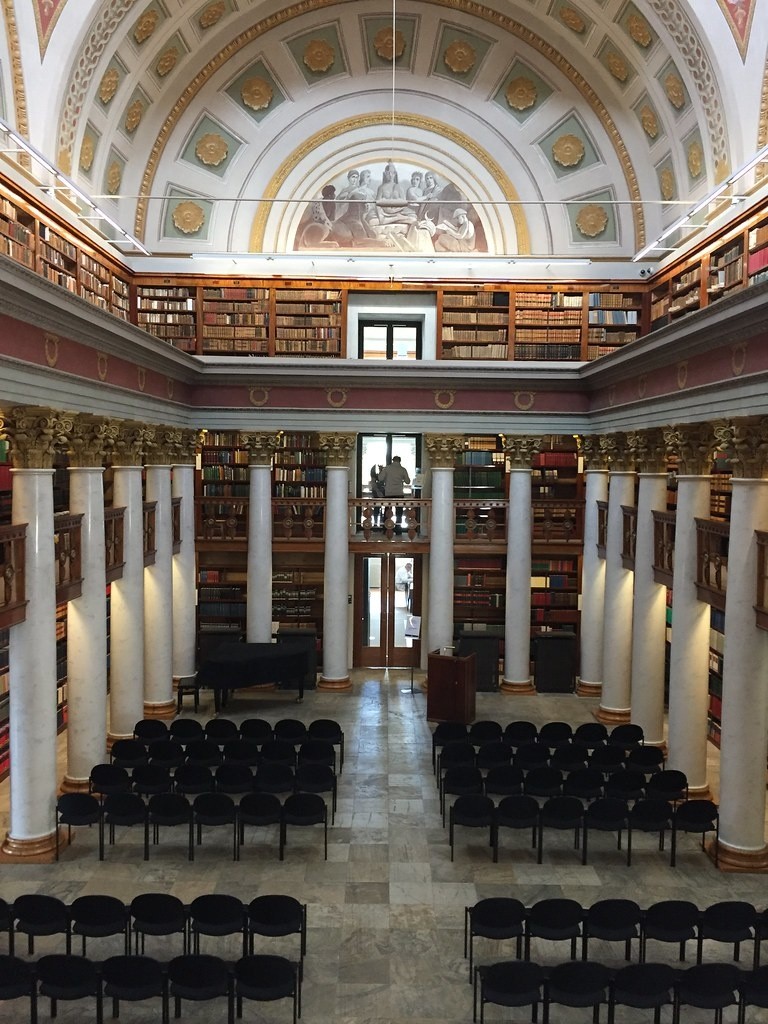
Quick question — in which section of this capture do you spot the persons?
[395,562,413,591]
[378,455,410,535]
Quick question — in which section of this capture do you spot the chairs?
[0,893,308,1024]
[55,720,341,862]
[433,722,718,868]
[464,897,768,1024]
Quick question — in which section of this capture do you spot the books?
[452,436,578,533]
[664,589,725,744]
[450,560,578,638]
[442,224,768,358]
[0,584,111,774]
[0,196,342,359]
[197,570,316,633]
[666,450,733,513]
[202,432,326,514]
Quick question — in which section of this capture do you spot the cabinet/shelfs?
[196,557,323,651]
[513,284,585,361]
[452,434,578,498]
[0,173,347,359]
[585,284,646,360]
[454,557,580,658]
[438,284,512,361]
[647,215,768,333]
[198,429,328,518]
